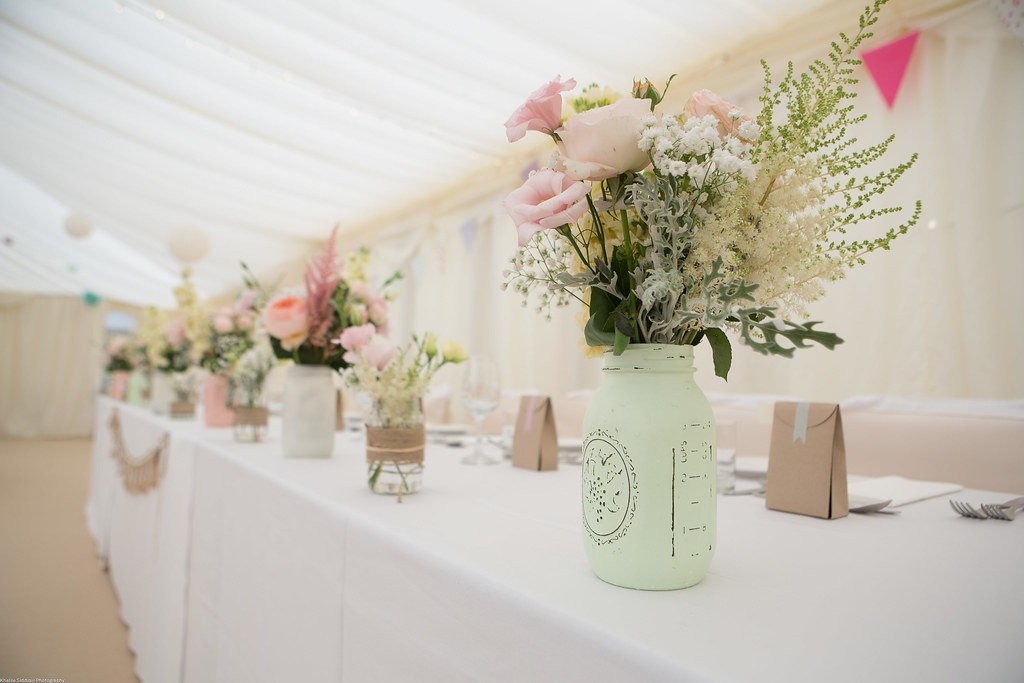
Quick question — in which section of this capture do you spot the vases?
[362,398,426,496]
[128,374,152,409]
[203,375,235,429]
[281,366,337,459]
[233,390,269,444]
[107,371,129,400]
[581,343,718,591]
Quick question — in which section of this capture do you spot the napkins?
[860,474,963,508]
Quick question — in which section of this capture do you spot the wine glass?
[456,356,498,465]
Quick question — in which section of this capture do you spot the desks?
[87,398,1024,682]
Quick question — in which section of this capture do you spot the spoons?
[848,498,892,515]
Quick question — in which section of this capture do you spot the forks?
[949,497,1024,519]
[981,500,1024,521]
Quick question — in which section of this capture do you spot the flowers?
[501,0,921,382]
[104,224,465,494]
